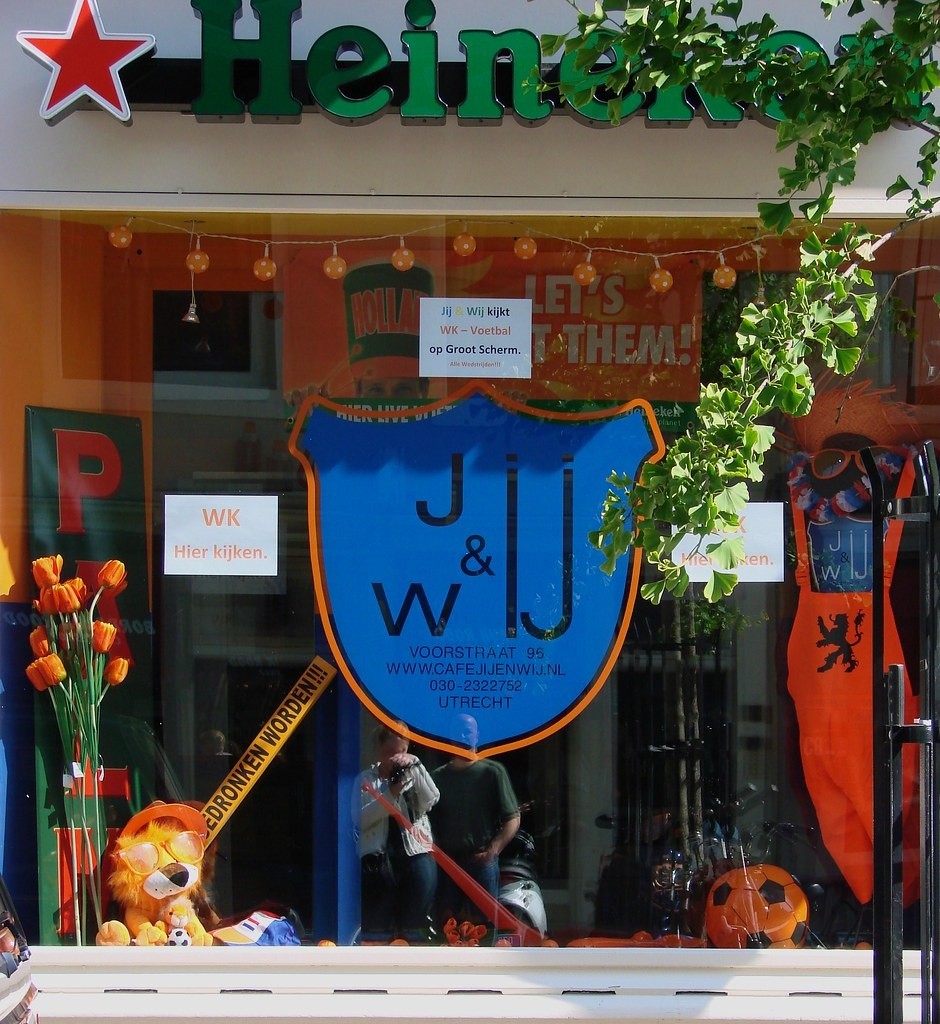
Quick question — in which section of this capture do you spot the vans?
[32,712,211,931]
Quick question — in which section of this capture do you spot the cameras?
[388,763,404,785]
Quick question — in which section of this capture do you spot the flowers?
[26,554,128,946]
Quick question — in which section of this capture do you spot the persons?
[428,713,521,947]
[350,720,440,946]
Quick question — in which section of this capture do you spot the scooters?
[480,796,555,947]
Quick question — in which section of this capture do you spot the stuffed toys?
[95,801,224,946]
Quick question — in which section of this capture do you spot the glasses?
[809,445,892,479]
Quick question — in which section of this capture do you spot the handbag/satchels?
[363,847,396,893]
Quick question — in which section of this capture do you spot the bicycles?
[655,821,823,952]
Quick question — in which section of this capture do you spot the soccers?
[704,863,810,948]
